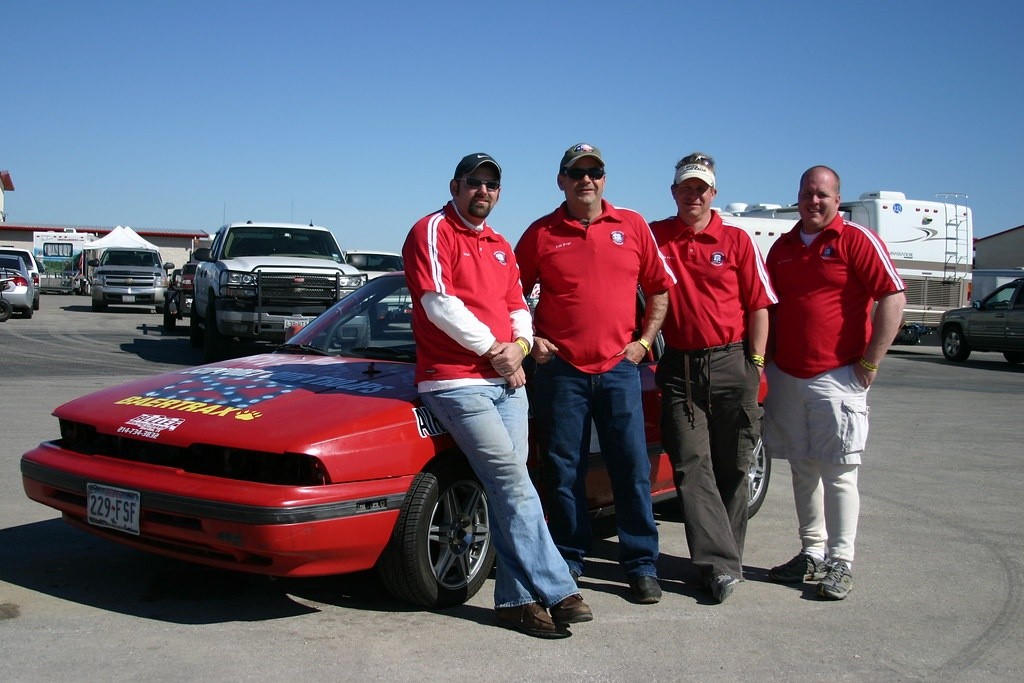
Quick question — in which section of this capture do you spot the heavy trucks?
[711,190,973,345]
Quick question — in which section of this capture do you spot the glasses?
[456,176,501,191]
[563,167,604,180]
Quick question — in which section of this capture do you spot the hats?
[454,152,502,183]
[560,143,605,172]
[674,164,715,189]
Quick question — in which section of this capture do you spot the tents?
[80,225,159,276]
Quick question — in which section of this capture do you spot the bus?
[32,227,109,296]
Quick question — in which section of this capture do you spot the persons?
[763,164,906,600]
[513,142,679,603]
[402,152,592,638]
[646,152,777,603]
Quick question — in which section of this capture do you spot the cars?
[20,269,773,610]
[0,254,36,320]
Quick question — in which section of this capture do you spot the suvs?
[0,245,45,311]
[939,278,1024,368]
[343,249,413,317]
[87,246,175,313]
[162,254,201,335]
[190,220,370,366]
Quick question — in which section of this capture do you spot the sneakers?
[569,568,579,584]
[769,552,828,581]
[710,573,738,602]
[628,573,661,604]
[494,601,568,638]
[549,593,593,624]
[815,561,854,598]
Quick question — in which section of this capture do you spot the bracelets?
[515,340,528,356]
[637,338,651,352]
[860,359,878,372]
[751,355,764,368]
